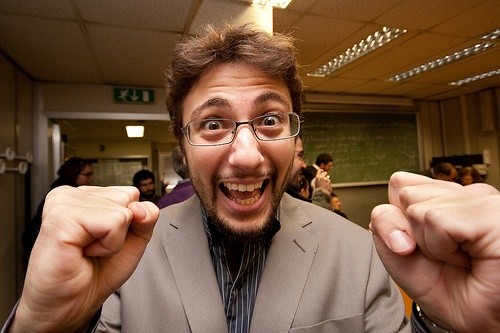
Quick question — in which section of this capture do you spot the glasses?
[78,172,94,177]
[182,112,305,146]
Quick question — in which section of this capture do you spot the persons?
[132,169,162,203]
[417,161,457,182]
[155,146,196,209]
[20,157,99,266]
[457,166,484,185]
[284,133,347,219]
[0,21,500,333]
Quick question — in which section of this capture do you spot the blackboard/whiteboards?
[300,111,425,188]
